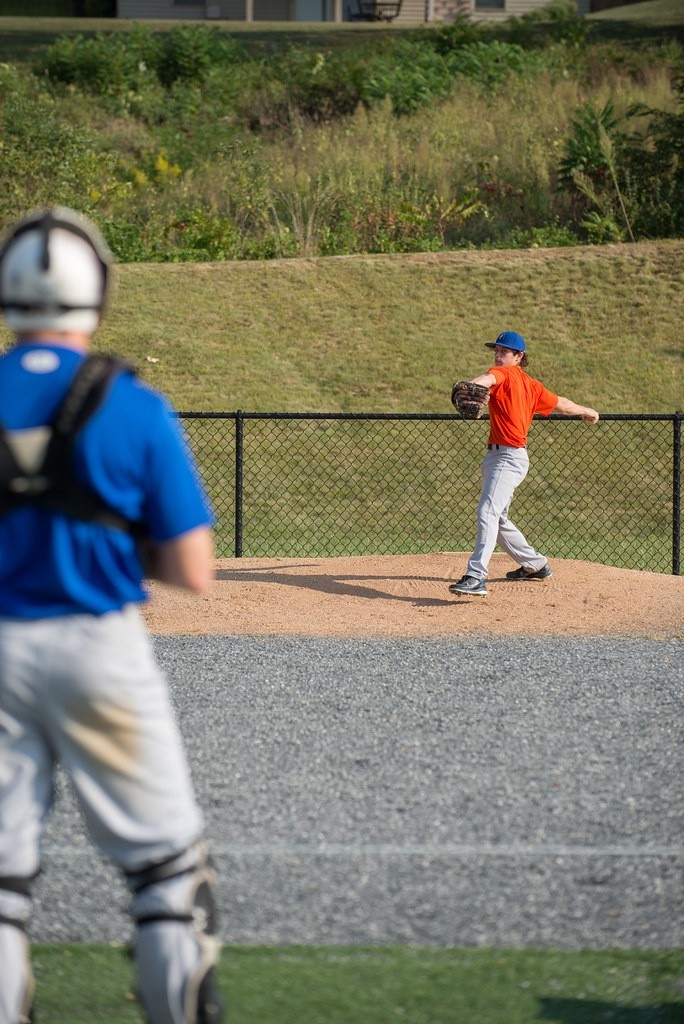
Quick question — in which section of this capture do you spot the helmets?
[0,206,108,329]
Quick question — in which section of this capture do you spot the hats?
[485,331,525,352]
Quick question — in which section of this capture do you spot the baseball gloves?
[450,380,492,420]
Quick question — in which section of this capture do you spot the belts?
[488,443,499,450]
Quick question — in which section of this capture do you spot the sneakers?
[449,575,487,598]
[506,563,552,579]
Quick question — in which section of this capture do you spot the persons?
[449,332,599,599]
[0,206,221,1024]
